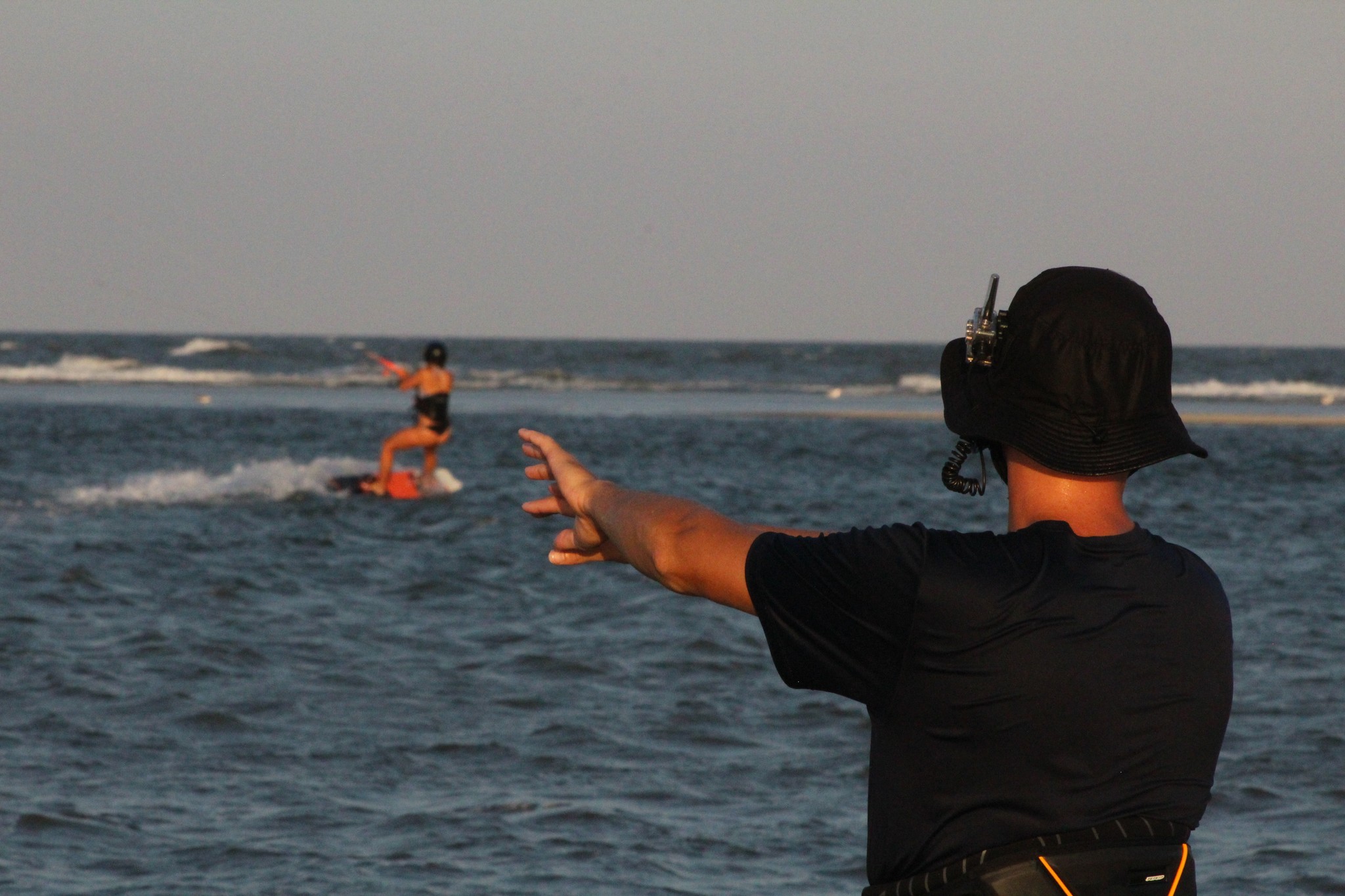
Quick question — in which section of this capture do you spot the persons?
[519,267,1234,896]
[360,342,452,496]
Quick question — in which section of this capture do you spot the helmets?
[422,341,446,364]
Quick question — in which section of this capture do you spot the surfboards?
[387,468,463,501]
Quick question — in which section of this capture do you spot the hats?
[938,263,1209,477]
